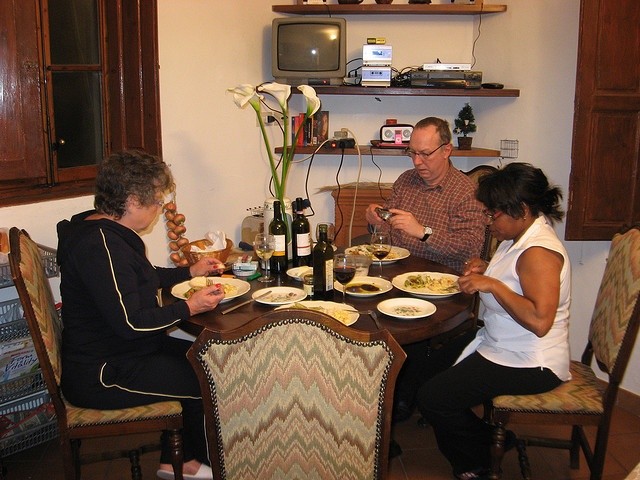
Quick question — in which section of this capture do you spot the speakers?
[380,124,414,142]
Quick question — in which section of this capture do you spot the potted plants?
[454,102,478,150]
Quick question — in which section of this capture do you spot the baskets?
[181,239,232,266]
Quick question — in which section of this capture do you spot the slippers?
[157,463,213,480]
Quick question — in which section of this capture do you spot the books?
[291,111,329,147]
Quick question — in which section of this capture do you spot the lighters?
[246,271,262,281]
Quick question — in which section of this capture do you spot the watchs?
[419,226,433,242]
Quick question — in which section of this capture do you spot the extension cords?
[344,74,361,85]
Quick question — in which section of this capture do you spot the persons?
[414,162,573,479]
[365,116,486,424]
[55,148,225,480]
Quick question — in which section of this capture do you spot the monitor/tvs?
[271,17,347,86]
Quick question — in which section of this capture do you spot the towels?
[190,230,228,252]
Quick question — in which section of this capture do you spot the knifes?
[368,309,384,331]
[221,290,272,316]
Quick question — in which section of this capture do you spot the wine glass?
[333,254,356,307]
[316,222,337,246]
[255,234,277,284]
[371,232,390,282]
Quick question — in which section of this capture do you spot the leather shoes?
[417,417,431,427]
[393,400,414,421]
[458,425,516,480]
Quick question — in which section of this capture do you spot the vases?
[264,197,295,262]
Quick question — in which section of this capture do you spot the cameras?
[375,207,395,223]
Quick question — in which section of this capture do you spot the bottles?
[269,201,288,276]
[313,224,334,301]
[292,197,312,269]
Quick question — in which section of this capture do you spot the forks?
[203,278,220,295]
[450,279,462,287]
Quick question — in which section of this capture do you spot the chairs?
[6,227,185,478]
[459,166,502,332]
[484,224,640,479]
[186,306,407,480]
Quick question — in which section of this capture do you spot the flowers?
[226,82,321,244]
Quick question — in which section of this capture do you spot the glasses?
[408,143,448,157]
[151,199,165,207]
[482,209,502,221]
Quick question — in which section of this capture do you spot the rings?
[464,261,468,265]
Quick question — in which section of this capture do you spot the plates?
[252,287,307,306]
[377,297,437,320]
[287,266,313,281]
[392,271,464,299]
[171,276,251,305]
[331,276,395,297]
[345,244,410,264]
[274,300,360,332]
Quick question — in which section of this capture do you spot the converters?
[326,139,354,148]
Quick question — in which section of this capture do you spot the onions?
[174,226,187,236]
[169,241,181,251]
[173,262,181,267]
[165,211,176,221]
[177,235,189,248]
[166,221,176,230]
[179,258,189,265]
[174,214,186,226]
[170,253,181,263]
[167,231,180,240]
[165,201,176,213]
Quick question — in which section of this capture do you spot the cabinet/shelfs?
[0,1,160,208]
